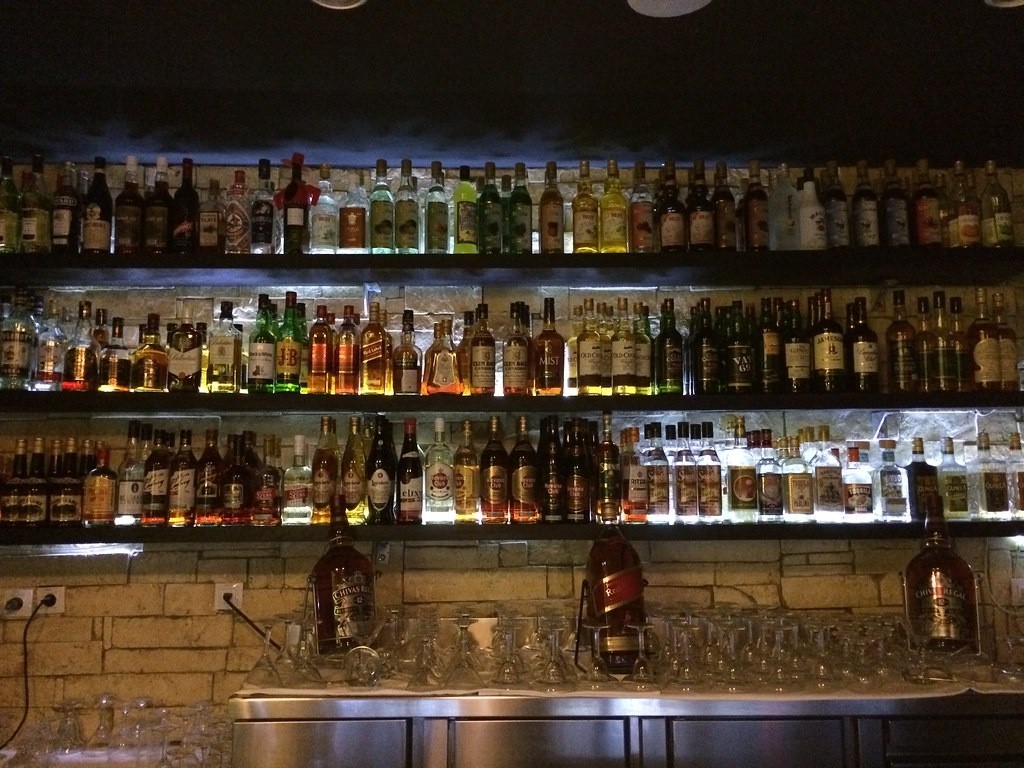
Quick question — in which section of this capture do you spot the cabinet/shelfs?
[233,713,1024,768]
[5,250,1023,543]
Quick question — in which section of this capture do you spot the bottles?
[903,496,984,658]
[965,432,1009,521]
[311,413,396,526]
[585,503,644,673]
[310,495,375,658]
[396,418,423,524]
[1004,432,1024,519]
[538,163,565,254]
[935,437,968,522]
[510,417,541,523]
[2,287,494,394]
[452,420,479,524]
[855,441,875,472]
[423,418,453,524]
[871,440,912,523]
[116,420,308,527]
[1,437,116,522]
[905,437,939,522]
[479,416,510,523]
[841,448,873,524]
[504,287,1024,395]
[963,439,977,467]
[1,152,533,253]
[538,410,844,525]
[571,158,1017,251]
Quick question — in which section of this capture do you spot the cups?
[996,636,1024,690]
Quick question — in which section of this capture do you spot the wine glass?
[243,614,283,689]
[265,613,304,689]
[583,623,613,682]
[287,621,328,690]
[51,697,234,768]
[628,601,934,691]
[343,602,577,690]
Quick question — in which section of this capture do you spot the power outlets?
[37,587,66,613]
[3,589,33,616]
[214,584,244,611]
